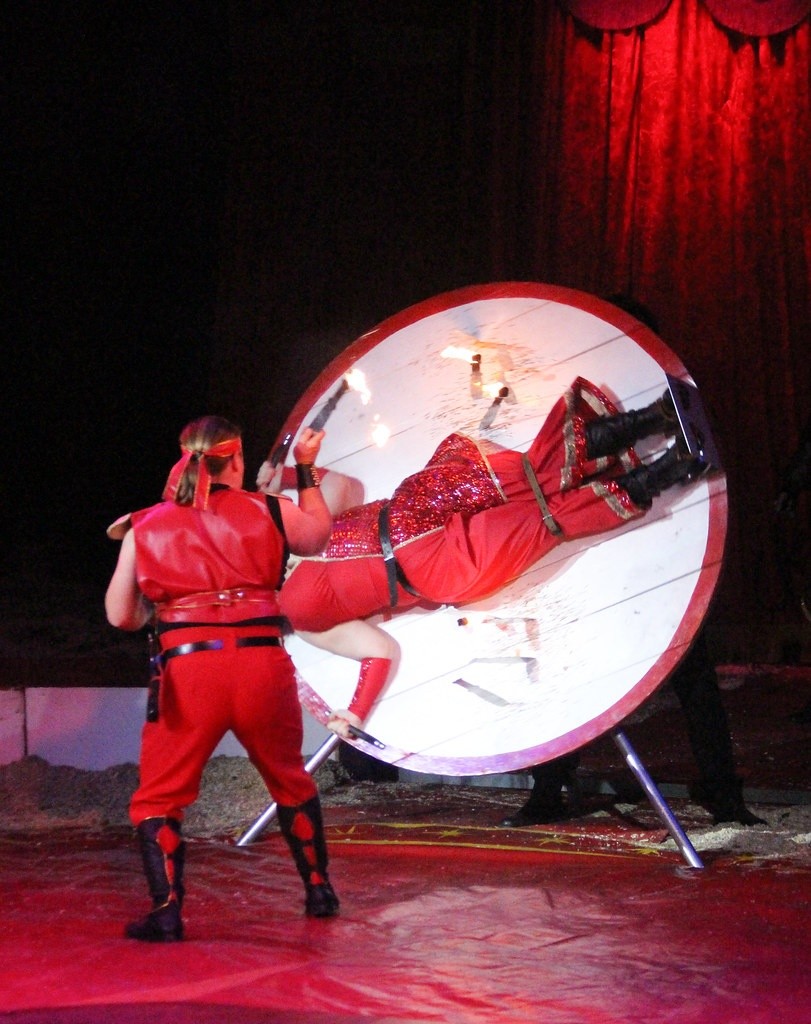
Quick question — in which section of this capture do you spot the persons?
[101,411,344,947]
[254,371,707,740]
[498,294,770,831]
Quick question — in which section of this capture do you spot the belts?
[522,451,564,540]
[378,500,422,607]
[162,636,282,661]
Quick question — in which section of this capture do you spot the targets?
[262,275,729,781]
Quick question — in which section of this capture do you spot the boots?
[583,388,681,460]
[612,426,715,509]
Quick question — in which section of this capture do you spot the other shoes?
[126,899,184,941]
[305,883,337,915]
[792,705,811,723]
[713,798,768,827]
[501,777,584,826]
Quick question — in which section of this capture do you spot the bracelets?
[296,463,320,491]
